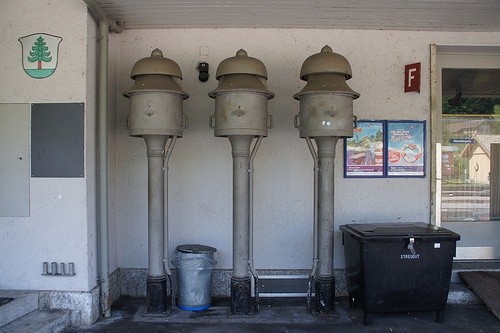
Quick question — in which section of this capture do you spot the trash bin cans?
[339,220,461,323]
[171,243,219,311]
